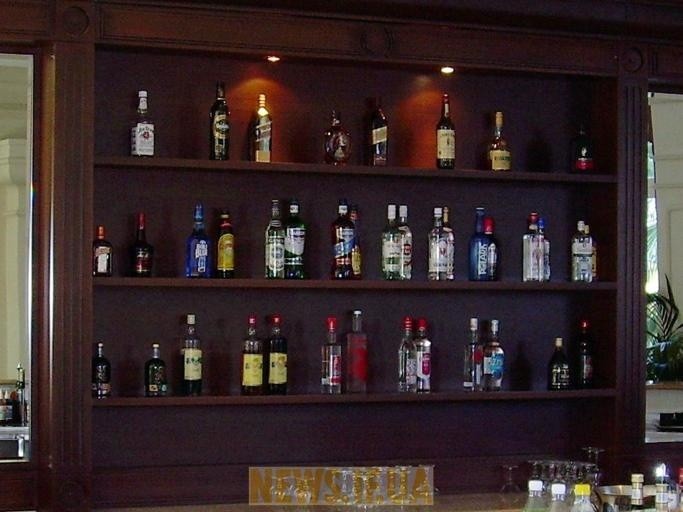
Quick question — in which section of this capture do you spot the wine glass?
[499,444,605,507]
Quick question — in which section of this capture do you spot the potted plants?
[641,272,682,412]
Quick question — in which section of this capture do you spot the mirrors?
[0,30,51,473]
[640,46,683,447]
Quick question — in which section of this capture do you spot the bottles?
[522,470,682,512]
[95,306,603,394]
[121,86,596,173]
[0,361,28,427]
[95,199,603,287]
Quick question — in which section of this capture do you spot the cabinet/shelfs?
[70,2,632,511]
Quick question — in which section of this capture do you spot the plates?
[654,425,682,432]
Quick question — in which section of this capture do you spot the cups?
[659,412,682,427]
